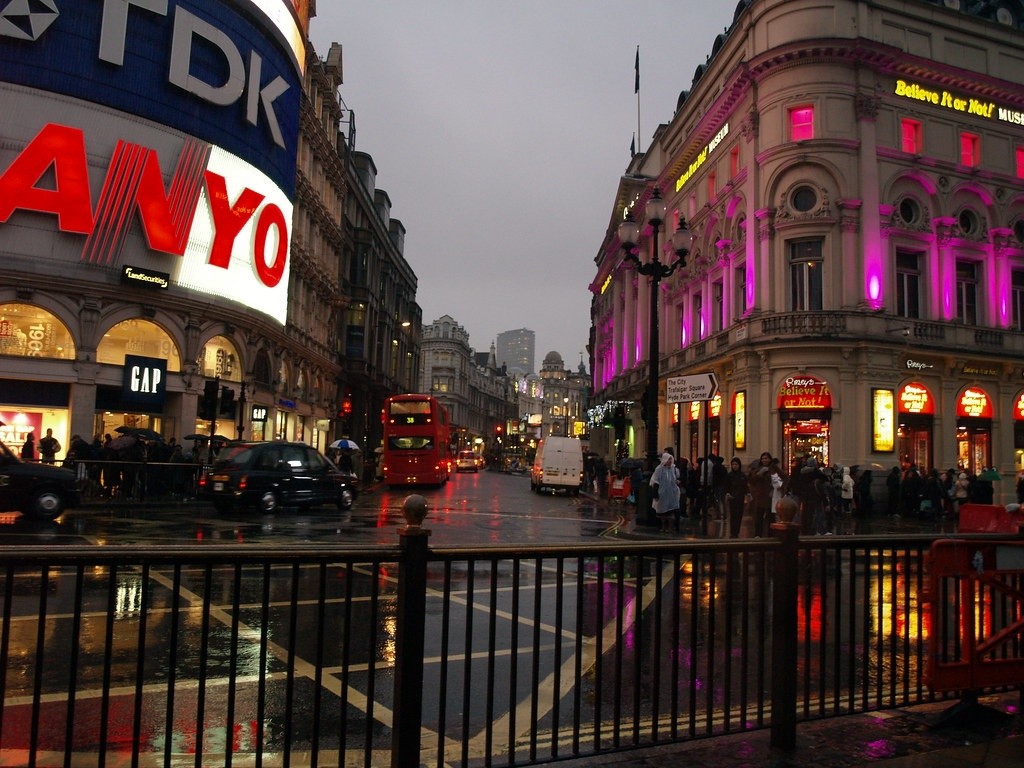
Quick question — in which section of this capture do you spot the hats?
[175,445,183,449]
[807,458,817,467]
[983,468,988,472]
[70,435,80,440]
[959,473,967,479]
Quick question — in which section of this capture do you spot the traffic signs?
[667,373,719,403]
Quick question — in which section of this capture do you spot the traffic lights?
[496,426,502,441]
[615,406,625,439]
[199,380,216,420]
[219,386,235,415]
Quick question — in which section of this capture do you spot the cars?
[616,457,645,474]
[456,450,478,473]
[495,454,526,474]
[198,440,358,515]
[0,441,83,520]
[476,454,485,470]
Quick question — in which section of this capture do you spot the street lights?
[619,186,693,526]
[564,398,569,437]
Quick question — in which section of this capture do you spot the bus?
[382,393,451,488]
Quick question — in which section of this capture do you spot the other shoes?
[814,532,821,536]
[824,532,832,535]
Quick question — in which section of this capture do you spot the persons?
[21,429,228,499]
[630,468,643,506]
[648,447,1024,539]
[337,451,354,475]
[329,439,360,455]
[584,456,608,492]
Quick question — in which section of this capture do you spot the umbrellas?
[128,427,165,441]
[184,434,209,441]
[616,457,649,469]
[208,435,230,441]
[114,426,137,433]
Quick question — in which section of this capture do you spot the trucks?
[530,436,584,496]
